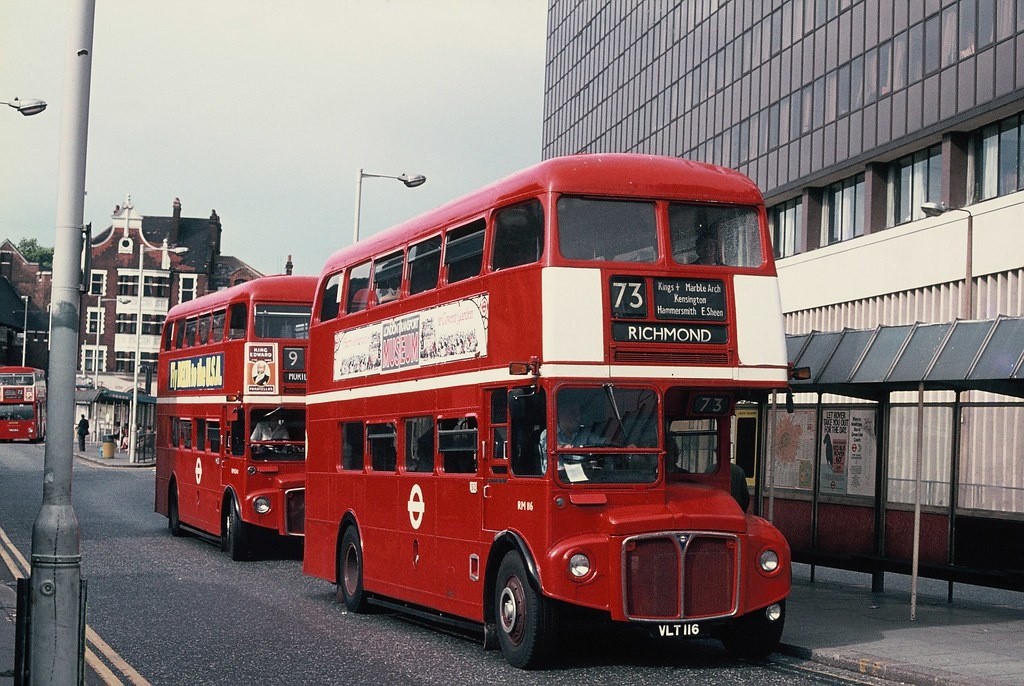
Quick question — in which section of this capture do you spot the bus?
[0,366,47,444]
[154,274,370,561]
[300,152,794,673]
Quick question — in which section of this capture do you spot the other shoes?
[121,446,123,448]
[125,449,128,451]
[124,446,126,449]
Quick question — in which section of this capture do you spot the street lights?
[93,297,131,442]
[354,168,427,243]
[129,244,190,463]
[921,202,974,508]
[21,295,29,367]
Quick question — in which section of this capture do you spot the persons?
[253,364,269,386]
[250,413,293,453]
[539,409,637,475]
[705,447,751,512]
[114,422,153,452]
[690,234,728,265]
[75,414,89,452]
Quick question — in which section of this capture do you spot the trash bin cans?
[103,434,115,459]
